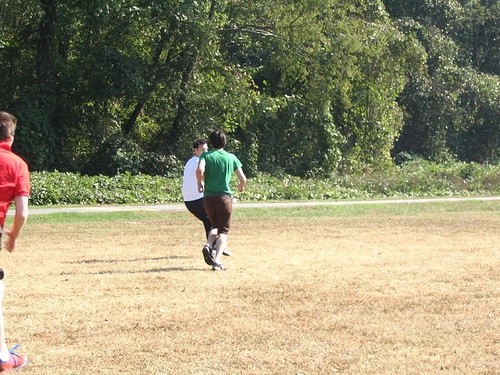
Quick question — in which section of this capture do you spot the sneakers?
[0,344,29,374]
[202,245,230,270]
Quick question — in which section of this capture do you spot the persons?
[0,111,30,373]
[182,139,233,257]
[196,130,247,271]
